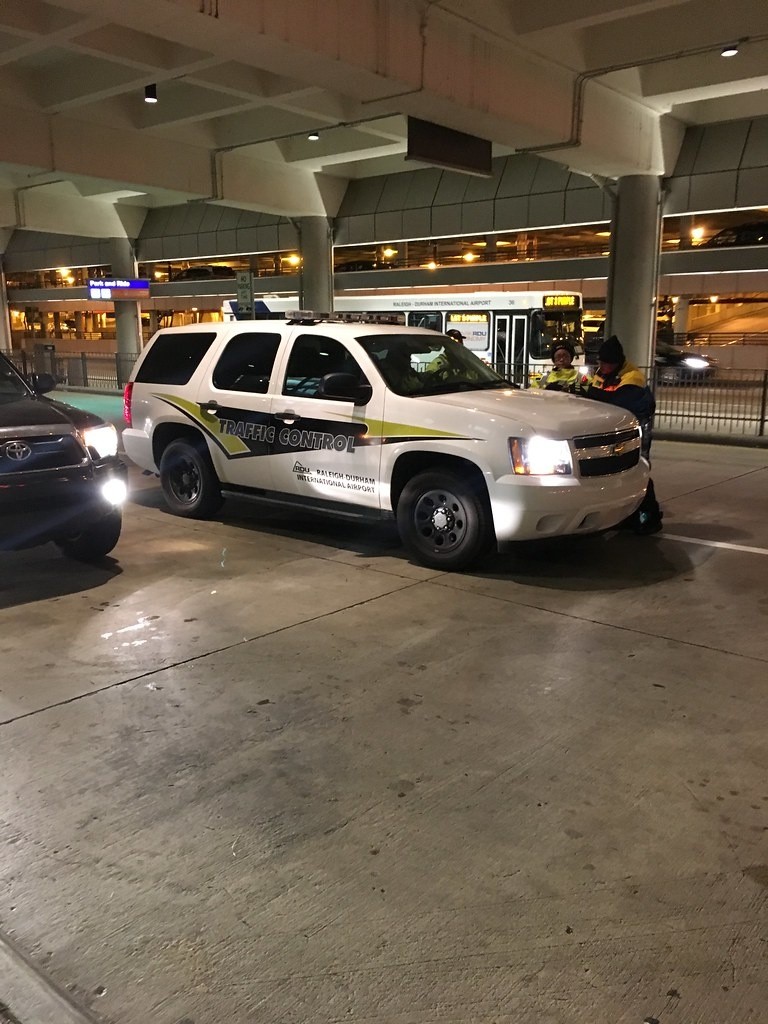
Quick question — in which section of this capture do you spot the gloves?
[569,383,586,396]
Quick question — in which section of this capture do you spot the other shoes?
[643,519,664,537]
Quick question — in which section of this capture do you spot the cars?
[690,221,768,249]
[0,349,130,563]
[333,259,393,273]
[169,265,235,282]
[581,316,719,386]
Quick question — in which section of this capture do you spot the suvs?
[118,318,652,572]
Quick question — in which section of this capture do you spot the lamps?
[308,133,318,140]
[145,84,157,102]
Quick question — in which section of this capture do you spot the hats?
[551,340,574,364]
[445,329,466,340]
[598,335,626,364]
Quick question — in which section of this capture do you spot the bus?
[216,288,586,395]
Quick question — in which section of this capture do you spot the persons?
[425,328,491,383]
[385,348,424,390]
[569,335,663,535]
[536,340,589,392]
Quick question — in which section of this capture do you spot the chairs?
[319,373,359,395]
